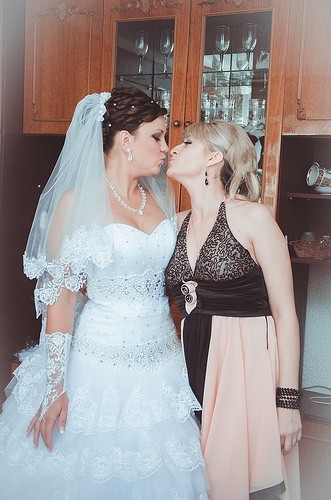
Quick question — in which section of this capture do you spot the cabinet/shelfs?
[23,0,331,265]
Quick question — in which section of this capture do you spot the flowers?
[181,276,198,315]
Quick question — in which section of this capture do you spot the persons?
[167,118,303,500]
[0,84,211,500]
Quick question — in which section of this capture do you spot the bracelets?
[276,386,303,410]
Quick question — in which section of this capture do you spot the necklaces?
[104,177,148,215]
[192,218,202,250]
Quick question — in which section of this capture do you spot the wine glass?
[236,48,250,85]
[211,50,223,88]
[215,26,230,71]
[242,22,257,70]
[133,31,149,80]
[159,29,174,80]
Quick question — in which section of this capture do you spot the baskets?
[290,240,331,257]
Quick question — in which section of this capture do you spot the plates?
[315,187,331,195]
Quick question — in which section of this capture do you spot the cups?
[156,90,171,125]
[200,94,250,124]
[249,100,266,136]
[306,165,331,187]
